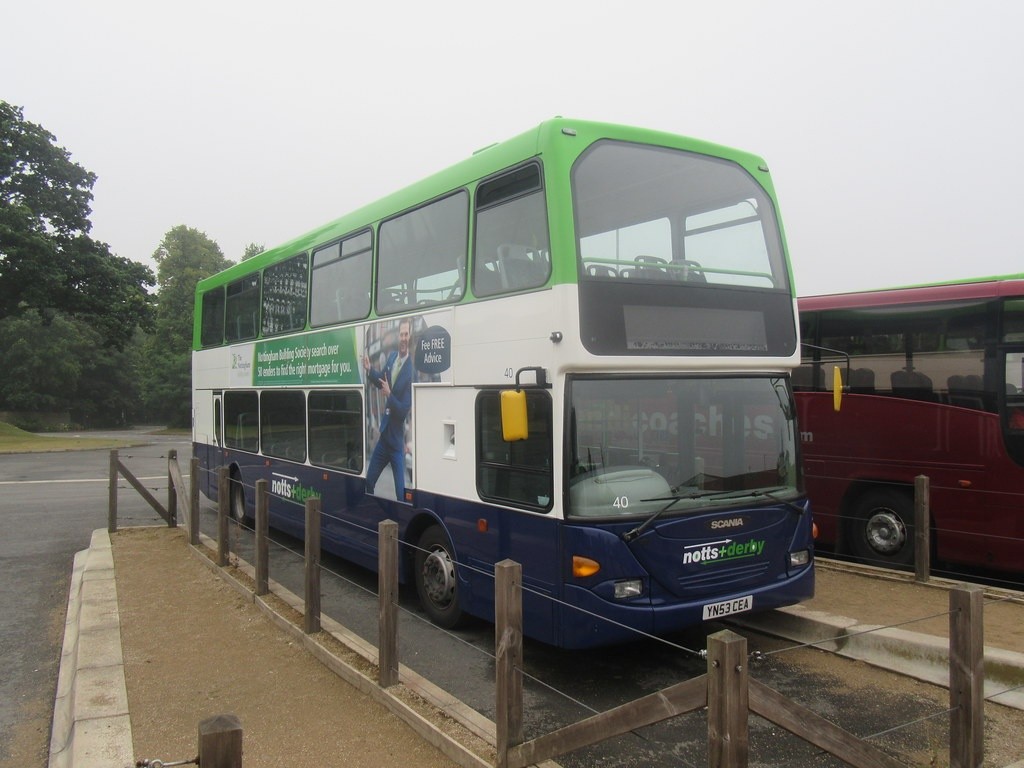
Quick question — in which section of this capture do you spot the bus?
[576,275,1023,582]
[188,116,816,653]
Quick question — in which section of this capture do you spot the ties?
[391,357,403,390]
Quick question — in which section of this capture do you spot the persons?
[362,318,417,503]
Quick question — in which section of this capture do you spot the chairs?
[210,242,1024,501]
